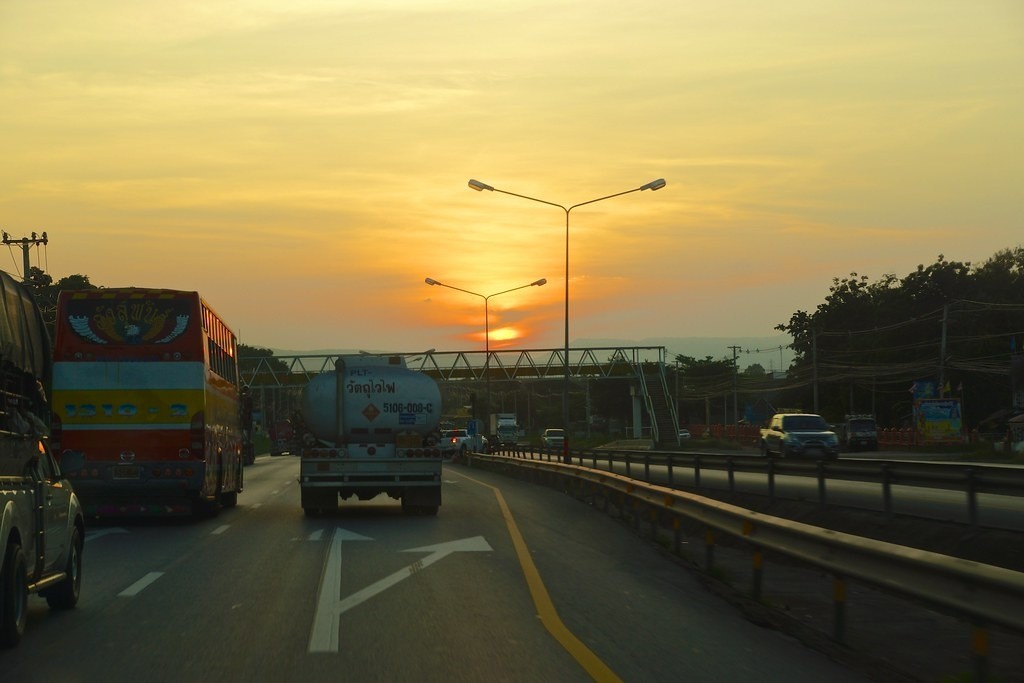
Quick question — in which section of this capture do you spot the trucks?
[489,413,519,443]
[1,269,51,477]
[845,414,877,450]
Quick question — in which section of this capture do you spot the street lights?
[424,275,548,415]
[468,177,667,464]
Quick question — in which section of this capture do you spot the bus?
[52,288,244,511]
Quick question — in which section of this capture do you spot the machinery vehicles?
[268,419,301,456]
[298,353,447,522]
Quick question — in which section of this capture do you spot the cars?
[679,428,691,439]
[542,428,565,447]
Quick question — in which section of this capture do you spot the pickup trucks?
[440,428,473,459]
[758,411,838,457]
[0,419,86,649]
